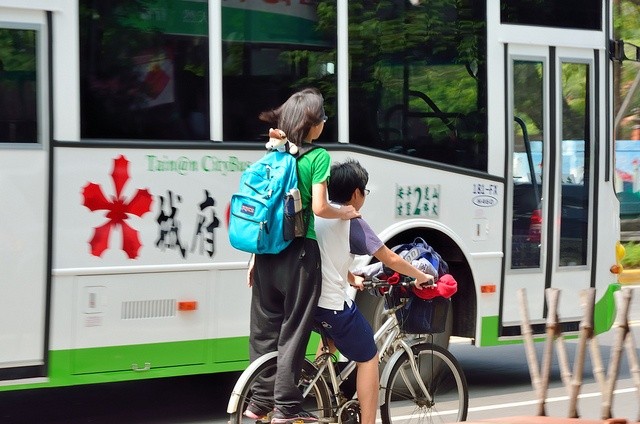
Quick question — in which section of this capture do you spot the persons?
[304,158,437,424]
[240,88,363,424]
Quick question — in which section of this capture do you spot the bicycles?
[227,279,469,423]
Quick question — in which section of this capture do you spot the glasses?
[364,188,370,196]
[322,116,328,122]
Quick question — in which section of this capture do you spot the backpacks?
[381,237,452,334]
[228,142,327,254]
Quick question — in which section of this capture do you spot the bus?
[0,0,637,391]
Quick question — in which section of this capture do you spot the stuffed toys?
[264,126,298,155]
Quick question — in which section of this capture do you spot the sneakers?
[243,404,267,419]
[271,408,319,424]
